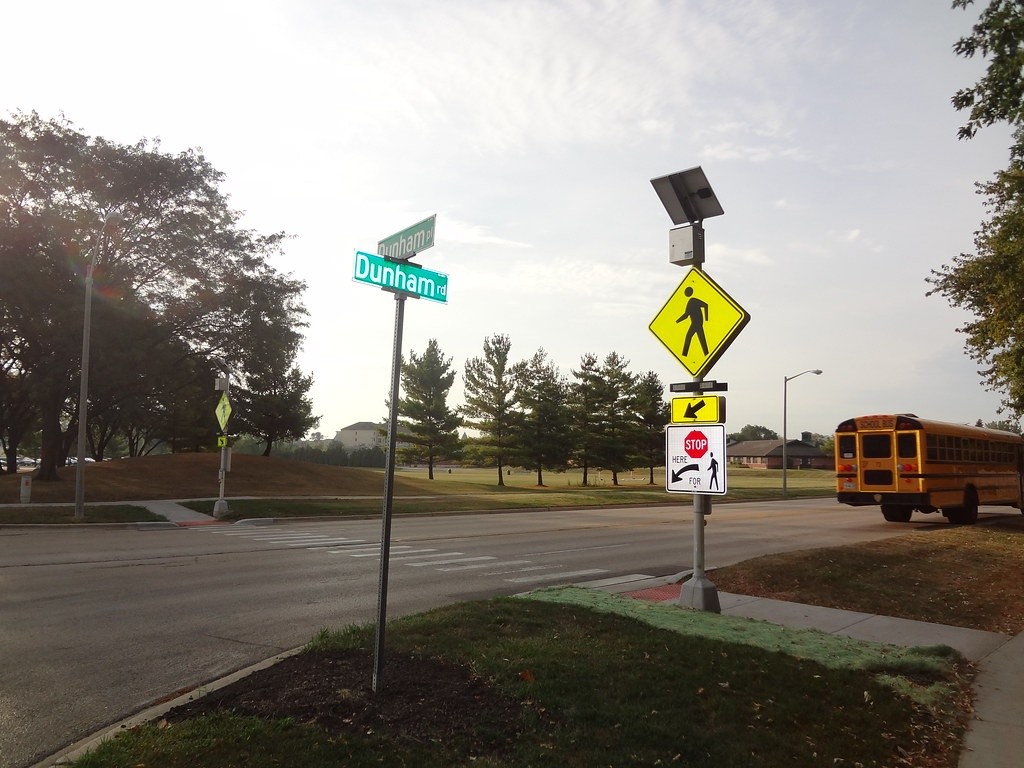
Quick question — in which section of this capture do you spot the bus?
[833,414,1023,523]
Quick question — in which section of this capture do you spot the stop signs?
[666,425,726,494]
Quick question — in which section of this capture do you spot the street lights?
[780,369,823,496]
[71,212,120,519]
